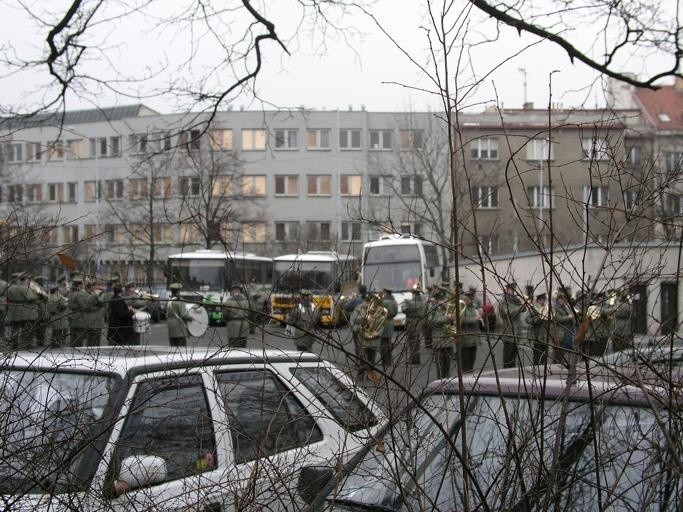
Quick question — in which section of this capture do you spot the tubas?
[443,293,467,336]
[515,291,620,320]
[365,291,388,339]
[135,290,160,303]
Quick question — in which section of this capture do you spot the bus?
[163,230,445,334]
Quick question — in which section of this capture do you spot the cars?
[136,283,170,320]
[291,359,683,511]
[586,344,683,370]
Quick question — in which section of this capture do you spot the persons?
[0,272,252,349]
[344,283,633,379]
[286,291,321,352]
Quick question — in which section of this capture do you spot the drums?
[132,312,150,333]
[186,303,208,337]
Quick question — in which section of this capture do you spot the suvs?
[0,342,392,512]
[462,290,498,332]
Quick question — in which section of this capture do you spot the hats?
[7,272,241,289]
[383,283,587,305]
[298,288,312,295]
